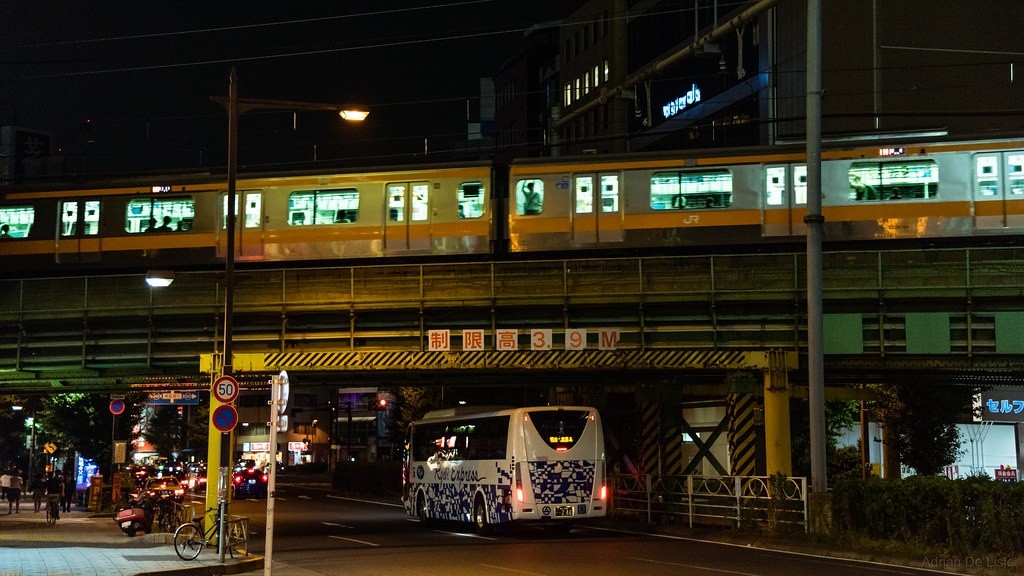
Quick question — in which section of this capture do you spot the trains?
[0,132,1024,274]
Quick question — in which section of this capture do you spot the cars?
[128,454,286,504]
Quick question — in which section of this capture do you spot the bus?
[401,404,609,538]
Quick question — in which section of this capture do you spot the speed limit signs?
[212,374,241,404]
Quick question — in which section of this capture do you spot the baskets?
[209,511,230,525]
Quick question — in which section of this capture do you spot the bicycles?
[172,506,233,561]
[42,495,59,525]
[111,489,181,537]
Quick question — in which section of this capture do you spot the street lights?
[10,404,37,487]
[209,65,374,558]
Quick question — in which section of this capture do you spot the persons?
[0,467,76,520]
[0,225,13,238]
[671,194,686,209]
[145,216,185,232]
[522,180,541,215]
[705,197,714,208]
[458,205,465,218]
[850,178,877,200]
[334,211,351,223]
[890,188,902,199]
